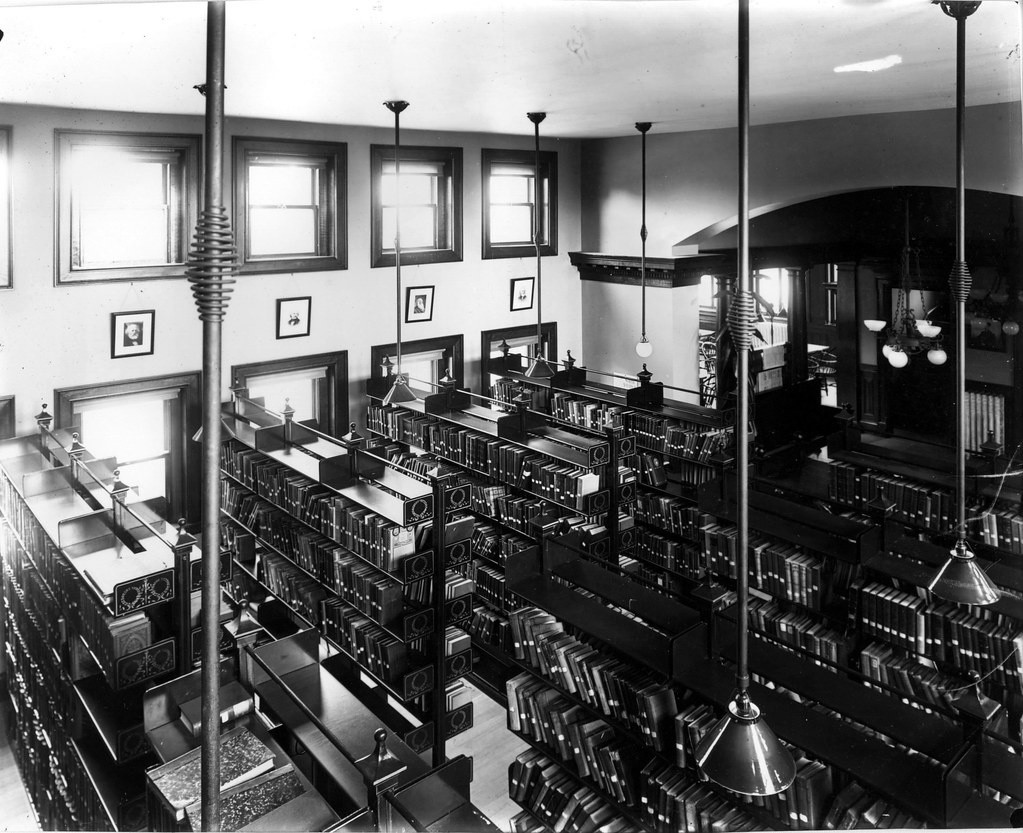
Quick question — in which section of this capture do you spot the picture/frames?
[510,276,536,311]
[110,309,155,359]
[277,296,311,339]
[405,285,435,323]
[966,304,1008,354]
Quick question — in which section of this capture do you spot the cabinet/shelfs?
[0,336,1023,833]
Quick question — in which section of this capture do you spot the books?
[191,378,722,714]
[179,681,255,738]
[506,453,1023,833]
[145,724,333,833]
[0,467,150,832]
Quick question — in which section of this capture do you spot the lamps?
[635,121,654,358]
[862,188,948,368]
[382,100,418,405]
[966,196,1023,335]
[926,0,1003,606]
[524,112,555,379]
[695,0,797,799]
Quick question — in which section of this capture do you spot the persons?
[288,310,300,327]
[124,324,143,347]
[518,289,526,300]
[414,298,425,313]
